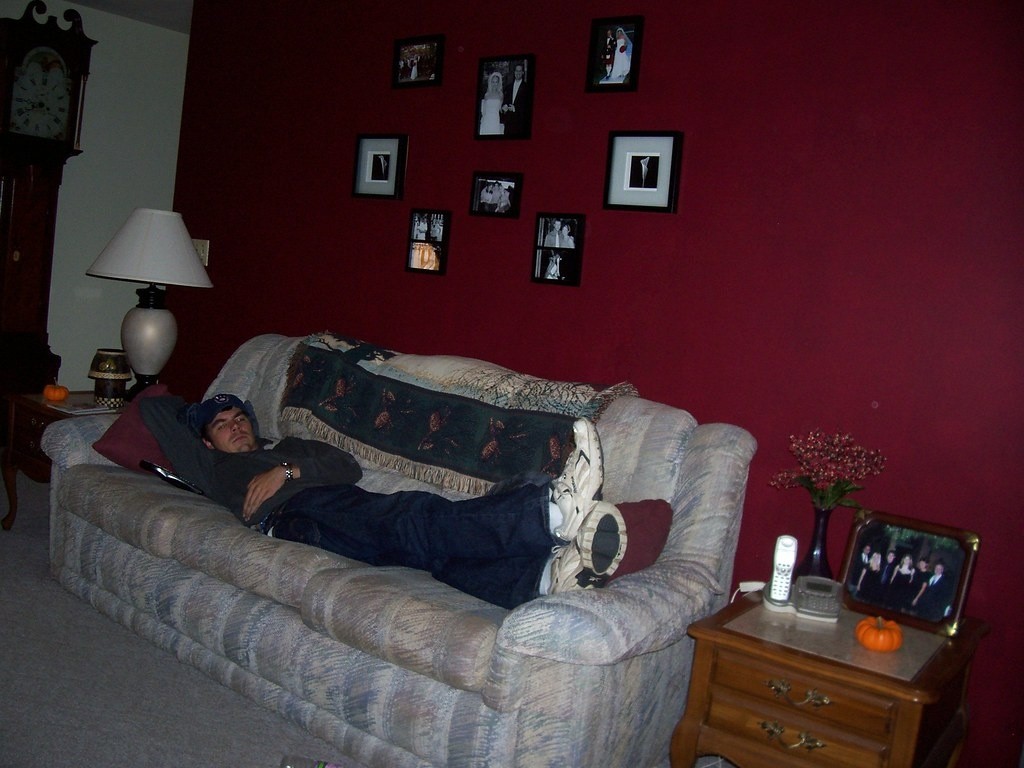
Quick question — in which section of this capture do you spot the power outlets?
[192,238,209,268]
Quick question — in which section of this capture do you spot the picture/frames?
[603,130,683,213]
[469,171,525,219]
[391,31,446,89]
[403,207,452,276]
[474,52,537,141]
[837,507,983,637]
[350,133,409,200]
[584,13,646,93]
[531,212,586,288]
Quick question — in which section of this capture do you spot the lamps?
[88,348,133,408]
[85,206,213,402]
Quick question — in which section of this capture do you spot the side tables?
[1,391,136,531]
[670,573,990,768]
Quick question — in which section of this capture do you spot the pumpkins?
[855,615,904,652]
[44,378,70,401]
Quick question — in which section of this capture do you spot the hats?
[197,394,248,426]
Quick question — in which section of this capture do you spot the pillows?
[605,499,673,586]
[90,383,176,476]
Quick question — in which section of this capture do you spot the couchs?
[39,333,757,768]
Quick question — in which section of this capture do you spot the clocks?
[7,48,73,141]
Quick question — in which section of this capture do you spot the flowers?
[767,424,888,522]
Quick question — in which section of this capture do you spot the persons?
[139,394,626,614]
[602,27,630,79]
[379,153,388,180]
[480,65,527,135]
[543,219,575,247]
[545,251,561,278]
[641,157,650,187]
[399,52,436,80]
[411,215,444,269]
[480,182,511,213]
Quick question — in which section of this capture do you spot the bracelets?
[279,459,295,484]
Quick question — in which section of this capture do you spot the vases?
[790,503,836,603]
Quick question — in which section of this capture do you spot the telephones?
[762,534,844,623]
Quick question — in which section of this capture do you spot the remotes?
[140,460,203,496]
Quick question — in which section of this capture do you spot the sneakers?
[553,416,604,540]
[547,501,627,594]
